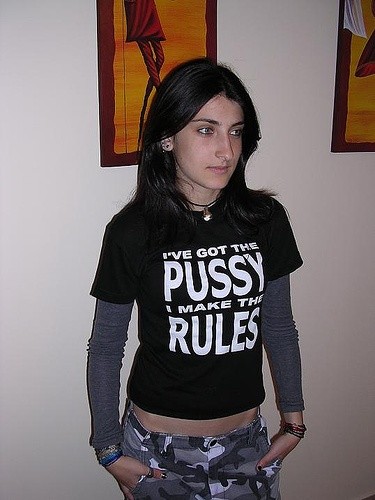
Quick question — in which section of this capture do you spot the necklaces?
[185,195,220,220]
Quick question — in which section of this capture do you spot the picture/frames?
[330,0,375,153]
[96,0,218,167]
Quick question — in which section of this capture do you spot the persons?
[84,57,308,500]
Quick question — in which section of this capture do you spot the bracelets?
[95,443,124,468]
[279,420,306,440]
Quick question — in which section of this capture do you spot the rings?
[146,466,155,478]
